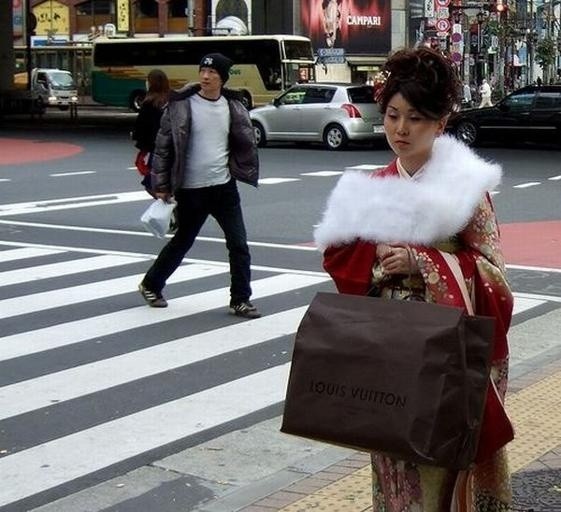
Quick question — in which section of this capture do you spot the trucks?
[6,67,81,115]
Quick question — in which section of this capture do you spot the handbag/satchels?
[281,292,496,470]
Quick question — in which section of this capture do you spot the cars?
[245,82,387,149]
[445,81,561,159]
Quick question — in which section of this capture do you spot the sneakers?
[230,301,261,318]
[139,284,168,307]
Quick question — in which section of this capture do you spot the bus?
[89,34,318,115]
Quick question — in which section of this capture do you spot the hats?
[199,53,233,84]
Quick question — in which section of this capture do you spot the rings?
[388,248,392,256]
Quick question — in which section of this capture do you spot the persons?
[504,73,511,89]
[136,51,262,319]
[476,77,494,109]
[462,81,476,110]
[307,44,517,512]
[132,67,174,204]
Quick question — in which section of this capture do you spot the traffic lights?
[483,4,509,13]
